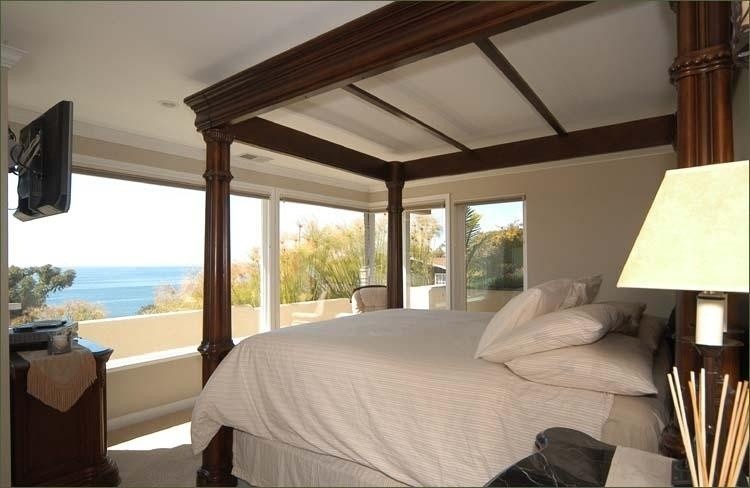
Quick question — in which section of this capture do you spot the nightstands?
[483,428,750,488]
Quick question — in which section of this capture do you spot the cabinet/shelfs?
[11,339,120,486]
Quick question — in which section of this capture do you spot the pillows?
[503,331,659,401]
[475,274,603,357]
[479,298,648,362]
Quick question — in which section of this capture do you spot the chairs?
[292,288,329,326]
[350,282,389,314]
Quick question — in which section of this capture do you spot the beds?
[183,0,750,487]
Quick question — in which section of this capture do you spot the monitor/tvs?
[12,100,74,222]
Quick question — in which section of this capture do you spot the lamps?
[617,158,749,456]
